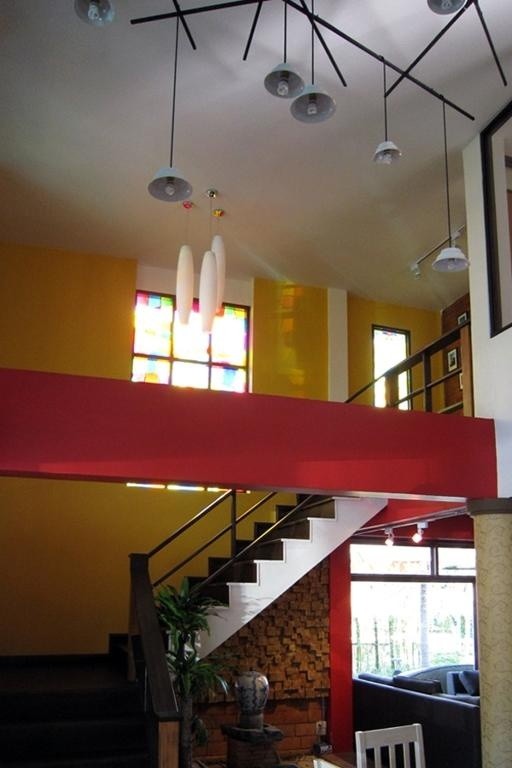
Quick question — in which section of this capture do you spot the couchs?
[352,673,481,768]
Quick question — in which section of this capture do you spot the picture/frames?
[446,347,459,372]
[458,370,463,391]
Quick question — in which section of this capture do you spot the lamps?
[412,521,428,543]
[384,527,395,548]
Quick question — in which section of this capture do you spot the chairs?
[409,665,480,705]
[354,721,427,768]
